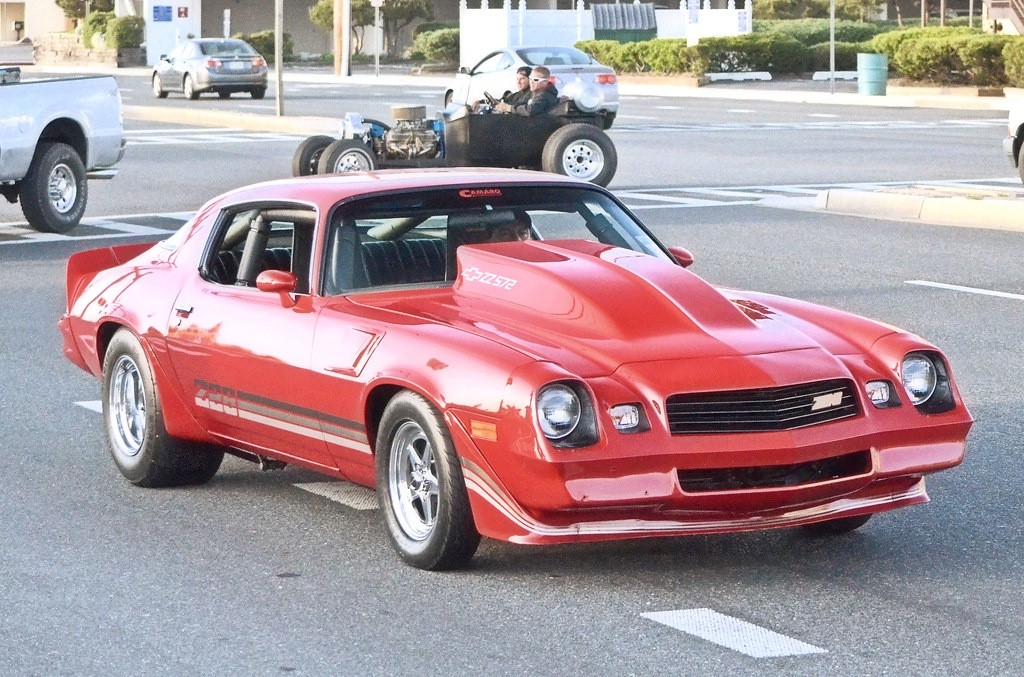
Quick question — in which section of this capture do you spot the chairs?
[544,56,565,65]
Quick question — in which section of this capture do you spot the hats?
[516,67,532,77]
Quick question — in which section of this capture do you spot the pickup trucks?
[0,75,128,229]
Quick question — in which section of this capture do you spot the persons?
[484,209,533,243]
[471,66,533,114]
[495,66,559,117]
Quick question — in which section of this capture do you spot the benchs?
[211,237,445,290]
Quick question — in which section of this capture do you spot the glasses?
[529,75,547,83]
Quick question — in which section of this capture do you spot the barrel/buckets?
[856,52,888,97]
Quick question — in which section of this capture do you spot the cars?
[151,38,269,100]
[444,45,618,130]
[59,167,973,572]
